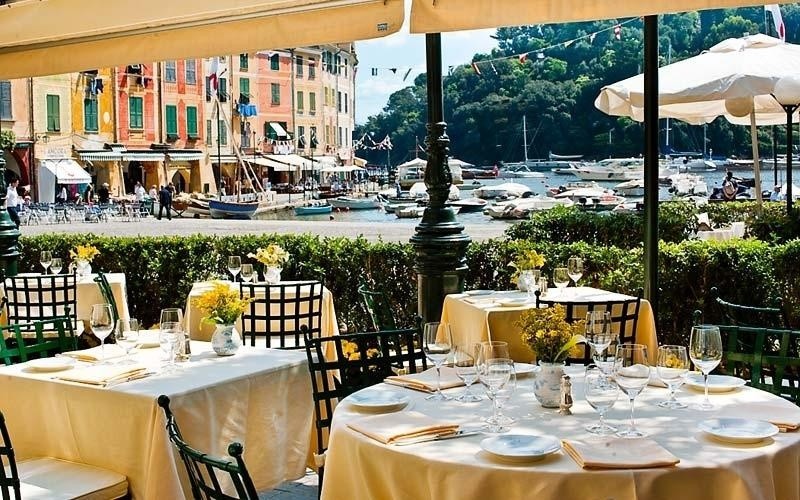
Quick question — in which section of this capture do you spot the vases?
[262,264,281,284]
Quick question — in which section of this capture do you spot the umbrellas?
[0,1,800,377]
[594,32,800,239]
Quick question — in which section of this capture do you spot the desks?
[687,221,745,239]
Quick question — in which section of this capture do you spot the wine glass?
[583,363,618,434]
[475,340,513,433]
[658,345,690,409]
[422,322,453,401]
[227,254,276,291]
[452,344,485,404]
[481,358,514,426]
[524,258,585,301]
[689,325,723,410]
[589,333,622,380]
[88,303,190,375]
[612,343,652,438]
[40,248,82,281]
[584,311,613,361]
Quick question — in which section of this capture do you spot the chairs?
[16,197,153,226]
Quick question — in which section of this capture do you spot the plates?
[466,290,494,296]
[480,362,539,378]
[347,391,410,408]
[479,435,564,463]
[28,357,77,371]
[697,417,779,444]
[493,299,528,307]
[689,374,745,391]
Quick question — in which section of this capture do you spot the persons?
[56,184,68,217]
[5,177,21,230]
[83,181,110,220]
[134,180,176,220]
[722,172,739,201]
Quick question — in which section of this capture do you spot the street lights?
[768,78,800,215]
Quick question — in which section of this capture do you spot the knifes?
[394,430,478,446]
[104,371,157,389]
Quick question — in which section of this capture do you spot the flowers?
[508,248,545,284]
[247,243,290,266]
